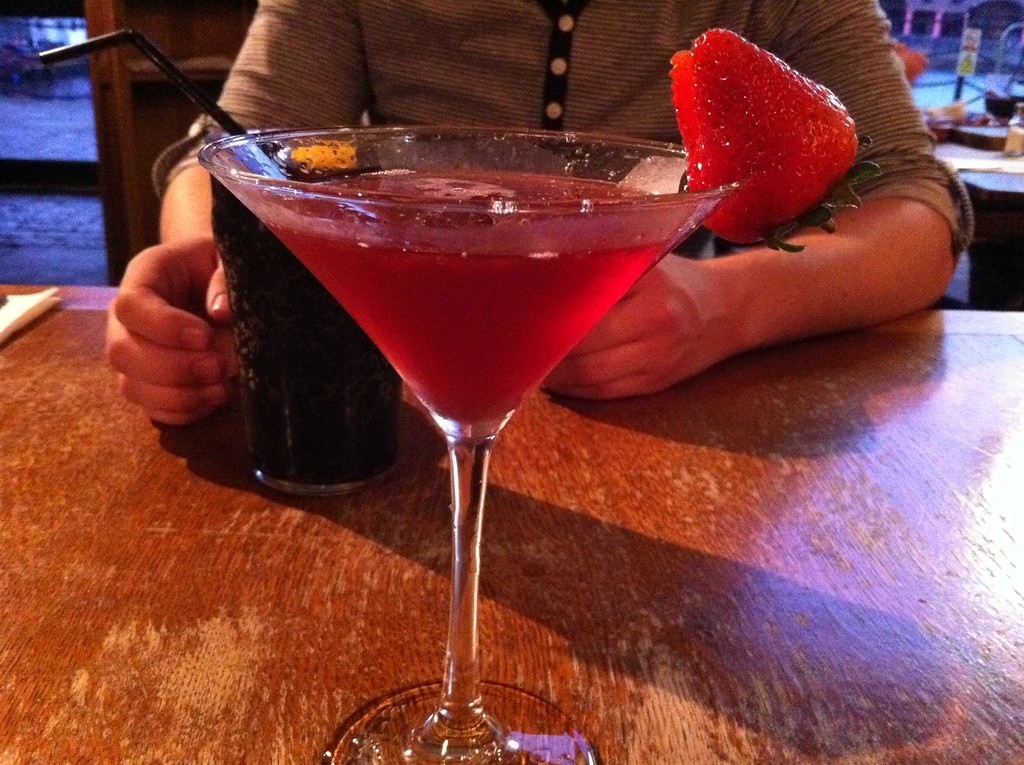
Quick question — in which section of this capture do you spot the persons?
[103,1,977,427]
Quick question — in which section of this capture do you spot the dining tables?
[931,140,1024,205]
[0,283,1022,765]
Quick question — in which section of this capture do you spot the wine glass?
[194,120,743,765]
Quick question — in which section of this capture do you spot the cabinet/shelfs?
[84,1,261,291]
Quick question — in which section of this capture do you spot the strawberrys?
[669,24,885,252]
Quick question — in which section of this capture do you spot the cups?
[211,175,406,494]
